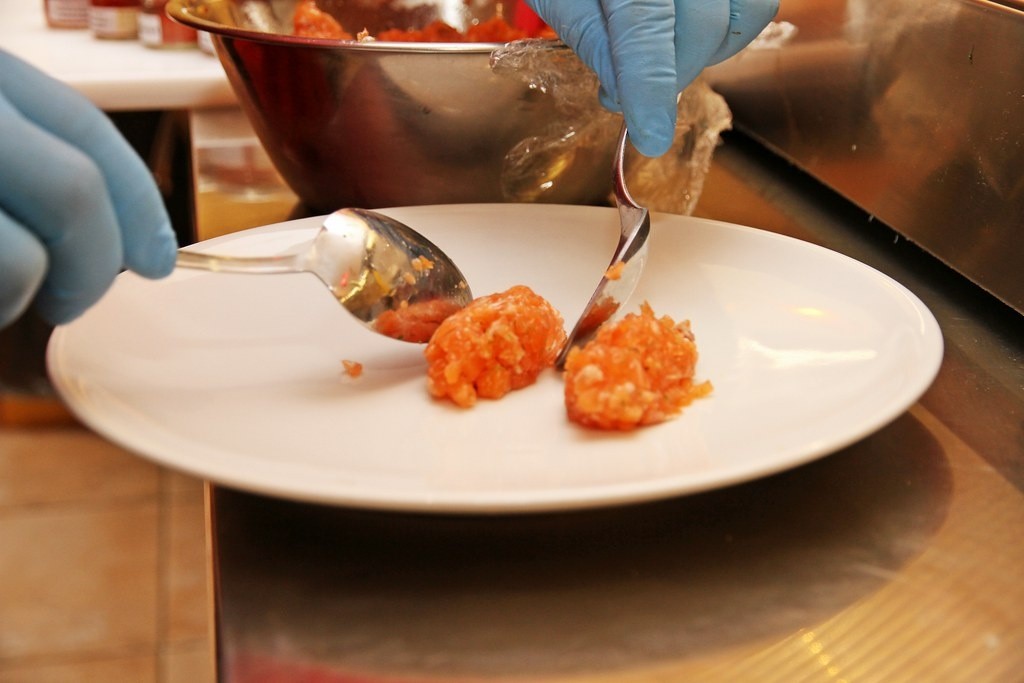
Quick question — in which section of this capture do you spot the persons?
[0,0,781,333]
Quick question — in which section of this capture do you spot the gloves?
[527,0,781,157]
[0,49,178,329]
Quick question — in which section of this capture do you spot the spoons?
[553,120,652,370]
[175,208,474,344]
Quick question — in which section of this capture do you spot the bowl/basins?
[163,0,625,207]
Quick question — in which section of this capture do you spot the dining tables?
[187,108,1024,683]
[0,0,235,111]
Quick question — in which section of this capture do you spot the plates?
[44,203,945,546]
